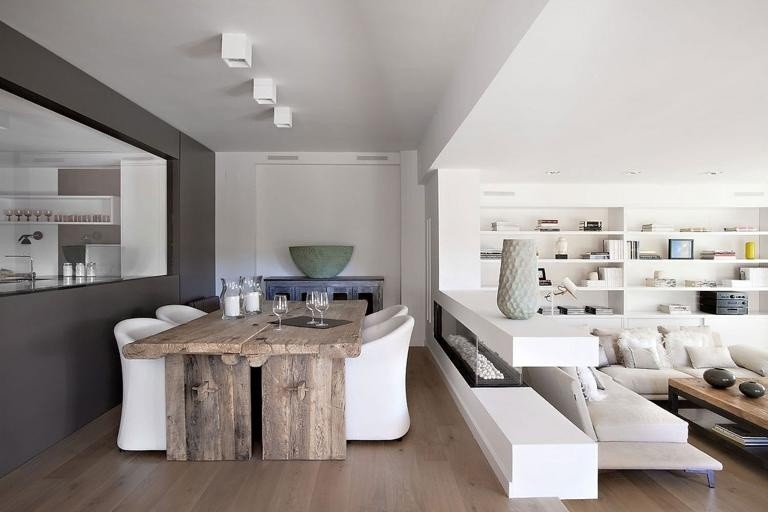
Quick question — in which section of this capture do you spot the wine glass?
[313,292,329,327]
[306,290,318,324]
[272,294,288,332]
[6,209,52,222]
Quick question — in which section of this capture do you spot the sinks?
[1,277,52,283]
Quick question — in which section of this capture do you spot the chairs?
[345,315,414,441]
[364,305,408,328]
[113,318,176,452]
[156,304,208,326]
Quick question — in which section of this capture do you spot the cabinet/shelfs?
[480,230,624,316]
[626,229,768,315]
[0,194,120,226]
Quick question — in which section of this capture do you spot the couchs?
[519,343,768,489]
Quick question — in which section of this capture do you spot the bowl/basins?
[288,245,354,279]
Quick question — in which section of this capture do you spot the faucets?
[5,253,34,277]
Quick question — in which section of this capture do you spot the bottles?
[739,271,745,280]
[63,262,73,278]
[238,274,265,315]
[75,262,84,276]
[220,278,245,321]
[554,237,568,256]
[86,261,95,278]
[746,242,755,260]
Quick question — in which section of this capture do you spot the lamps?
[220,32,293,130]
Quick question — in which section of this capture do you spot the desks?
[122,299,369,462]
[264,276,384,316]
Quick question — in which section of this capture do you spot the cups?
[654,271,664,280]
[587,271,599,282]
[53,214,109,222]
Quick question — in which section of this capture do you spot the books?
[645,276,677,288]
[680,226,712,232]
[581,239,662,260]
[533,220,561,231]
[539,305,614,314]
[539,279,552,286]
[491,222,520,231]
[641,223,676,232]
[579,220,602,231]
[724,226,757,232]
[700,249,737,261]
[581,266,623,289]
[711,422,768,448]
[684,279,717,288]
[480,248,503,259]
[657,303,689,315]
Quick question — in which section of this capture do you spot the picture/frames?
[668,239,693,259]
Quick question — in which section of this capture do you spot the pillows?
[571,326,738,400]
